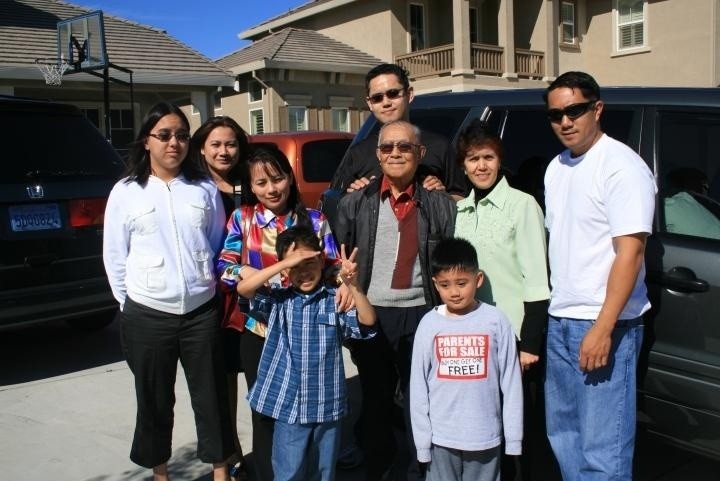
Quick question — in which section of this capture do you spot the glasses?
[369,88,404,104]
[150,132,190,142]
[548,102,591,122]
[379,143,420,154]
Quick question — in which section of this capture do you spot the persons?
[333,65,467,203]
[101,100,236,481]
[193,116,259,477]
[219,144,356,480]
[453,117,551,374]
[334,120,457,481]
[406,237,526,481]
[543,73,658,480]
[237,226,376,481]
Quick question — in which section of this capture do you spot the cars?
[231,129,356,210]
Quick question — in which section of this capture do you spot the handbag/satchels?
[221,287,245,332]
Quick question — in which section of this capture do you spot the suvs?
[301,84,719,473]
[1,97,141,331]
